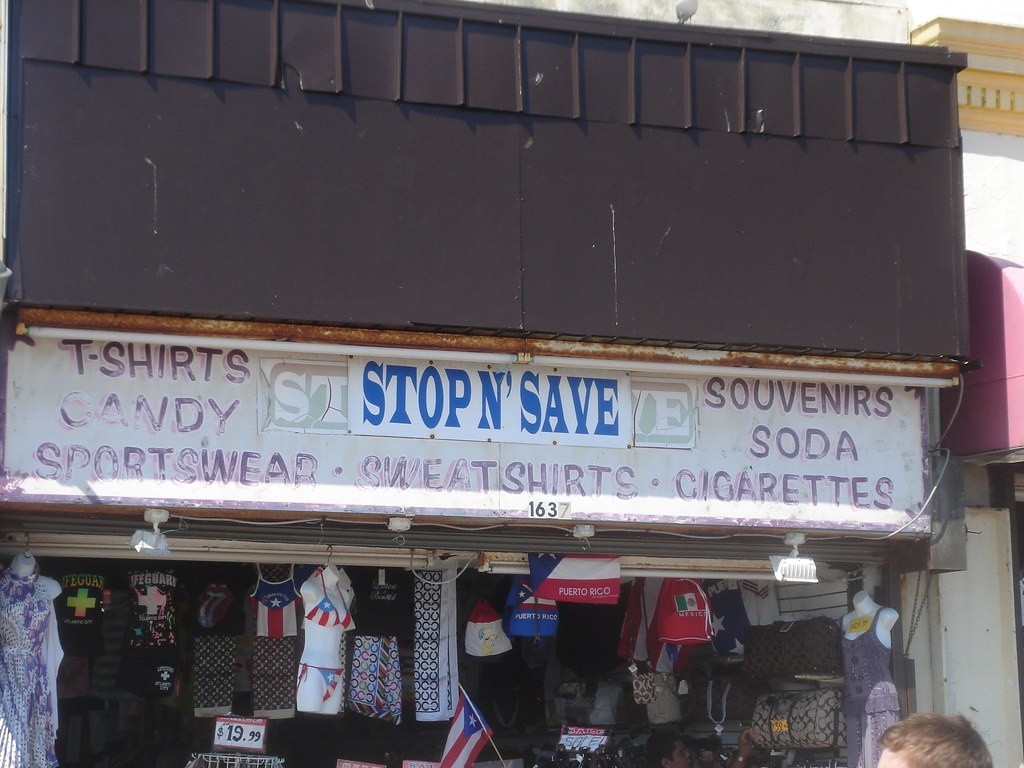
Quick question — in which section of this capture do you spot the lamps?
[677,0,698,24]
[769,532,819,584]
[129,509,170,553]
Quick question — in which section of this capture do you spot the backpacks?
[502,574,560,645]
[656,578,714,645]
[464,599,515,671]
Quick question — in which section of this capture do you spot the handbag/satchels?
[527,551,621,603]
[457,664,680,738]
[793,760,847,768]
[750,688,849,749]
[743,616,842,675]
[682,676,744,724]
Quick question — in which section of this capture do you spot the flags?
[438,689,494,768]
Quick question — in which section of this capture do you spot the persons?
[647,729,756,768]
[874,711,995,768]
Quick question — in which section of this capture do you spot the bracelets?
[733,753,750,764]
